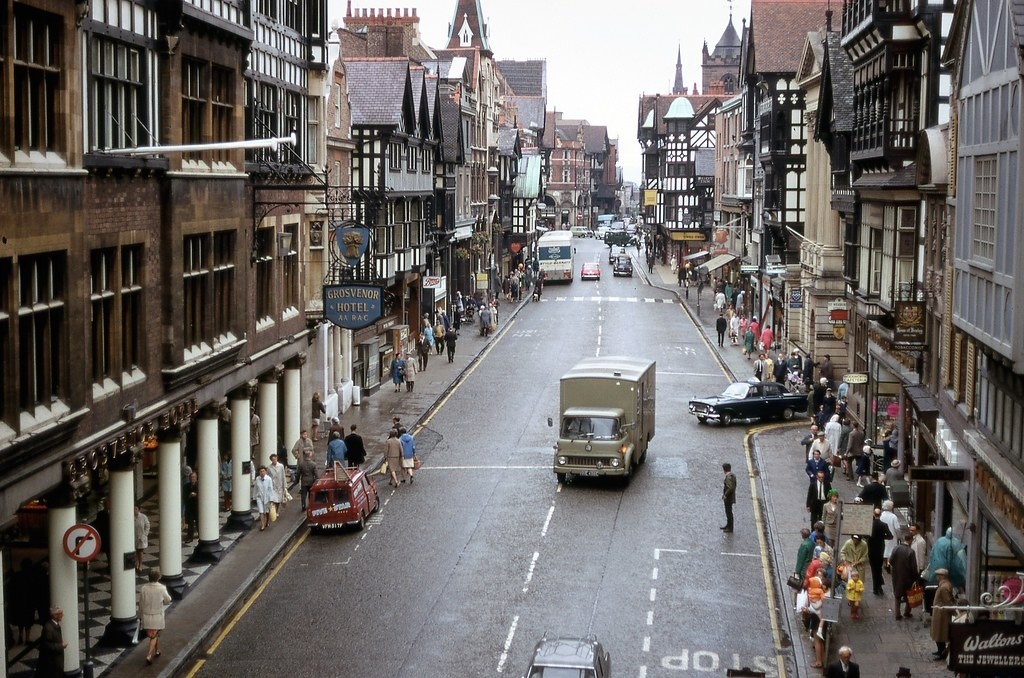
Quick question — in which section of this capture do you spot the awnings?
[683,251,708,260]
[694,254,735,274]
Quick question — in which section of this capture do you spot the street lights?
[685,263,690,299]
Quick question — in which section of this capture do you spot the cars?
[688,382,809,427]
[520,632,611,678]
[613,254,633,277]
[597,214,638,234]
[570,226,594,238]
[593,227,610,239]
[608,246,625,264]
[581,262,600,280]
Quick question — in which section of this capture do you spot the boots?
[816,627,825,641]
[856,476,871,488]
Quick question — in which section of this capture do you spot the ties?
[819,482,821,502]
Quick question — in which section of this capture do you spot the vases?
[456,257,467,264]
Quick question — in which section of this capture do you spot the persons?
[251,454,287,530]
[291,431,320,511]
[672,255,774,359]
[97,498,110,570]
[138,571,172,665]
[391,256,542,393]
[720,463,736,533]
[134,501,150,573]
[250,407,260,459]
[754,348,973,678]
[6,558,36,646]
[634,223,654,273]
[37,605,68,678]
[384,416,416,487]
[182,466,199,542]
[327,417,366,468]
[220,449,232,512]
[312,392,325,441]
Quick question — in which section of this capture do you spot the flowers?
[470,244,484,255]
[472,231,490,244]
[453,247,470,262]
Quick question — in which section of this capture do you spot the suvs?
[604,231,636,248]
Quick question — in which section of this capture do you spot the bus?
[536,230,576,283]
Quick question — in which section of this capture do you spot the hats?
[406,352,412,355]
[891,459,901,468]
[823,354,830,361]
[934,568,950,576]
[851,534,861,541]
[817,430,825,436]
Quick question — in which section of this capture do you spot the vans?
[306,460,380,531]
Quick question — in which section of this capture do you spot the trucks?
[547,357,658,484]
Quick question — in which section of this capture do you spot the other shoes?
[720,526,733,532]
[809,662,824,668]
[185,538,193,543]
[260,521,271,530]
[850,587,914,622]
[388,475,415,489]
[931,651,944,660]
[145,650,162,665]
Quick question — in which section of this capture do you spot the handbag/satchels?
[728,328,737,338]
[905,580,923,608]
[397,366,405,378]
[283,490,293,504]
[380,460,389,474]
[742,345,747,355]
[268,502,277,522]
[412,456,421,470]
[787,572,803,591]
